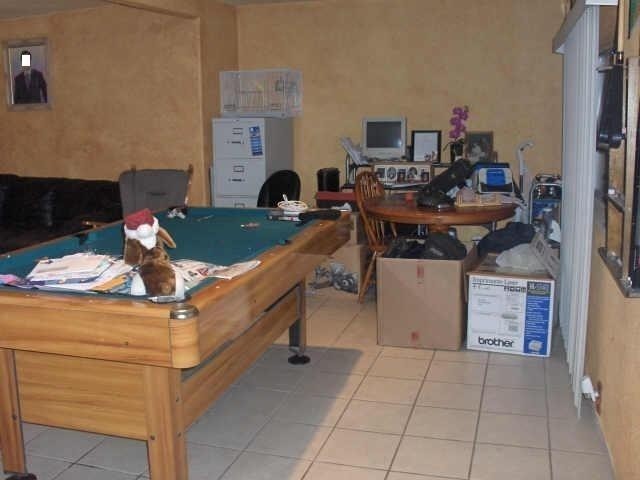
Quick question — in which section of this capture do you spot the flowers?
[443,102,470,152]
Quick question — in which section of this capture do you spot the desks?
[359,186,522,240]
[0,201,357,478]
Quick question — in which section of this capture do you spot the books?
[19,252,141,294]
[183,279,199,290]
[266,210,301,222]
[170,259,261,279]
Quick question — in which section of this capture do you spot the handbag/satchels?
[382,232,467,260]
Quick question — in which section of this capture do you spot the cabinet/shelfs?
[206,110,297,210]
[526,170,565,236]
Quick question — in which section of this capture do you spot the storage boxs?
[301,241,370,298]
[464,248,561,363]
[371,231,479,355]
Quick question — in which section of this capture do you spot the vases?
[449,142,463,164]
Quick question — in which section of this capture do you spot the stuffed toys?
[124,210,185,297]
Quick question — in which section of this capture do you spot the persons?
[376,168,428,183]
[15,50,47,102]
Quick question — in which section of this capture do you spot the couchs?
[0,170,121,260]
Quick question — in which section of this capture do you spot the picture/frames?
[4,35,56,115]
[410,126,442,168]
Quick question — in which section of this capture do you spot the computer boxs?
[318,167,339,207]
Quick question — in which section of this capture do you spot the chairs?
[255,168,303,212]
[354,166,403,306]
[313,166,359,215]
[117,157,196,226]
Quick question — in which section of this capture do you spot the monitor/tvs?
[360,116,407,162]
